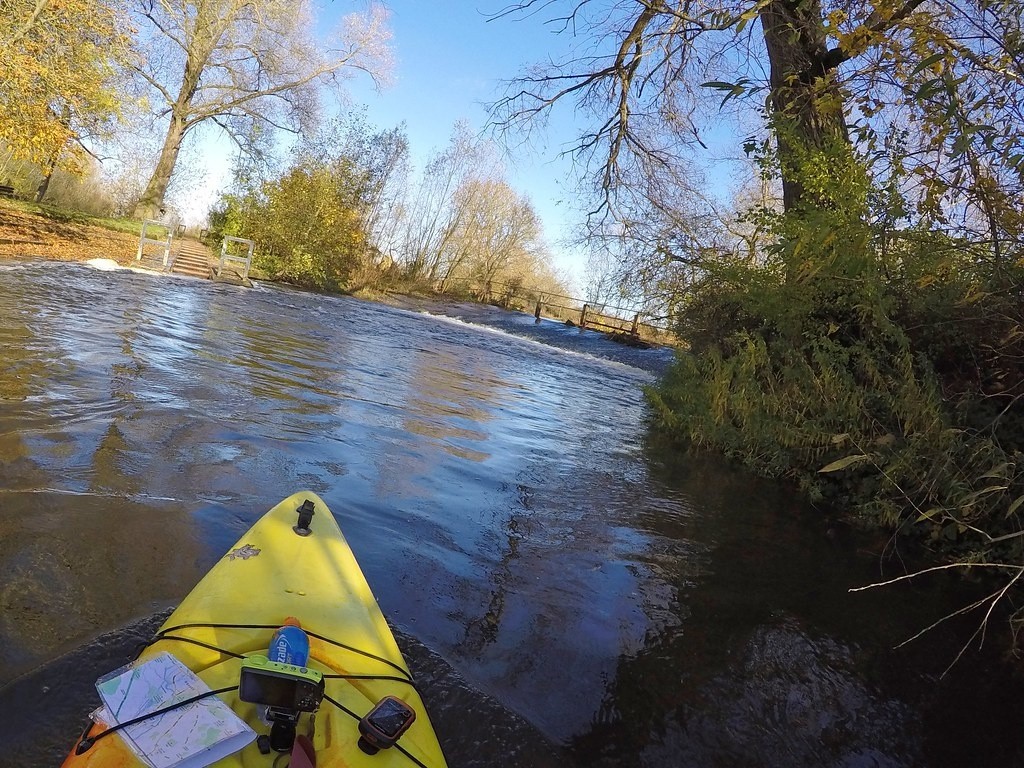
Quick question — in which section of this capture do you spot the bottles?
[255,617,309,728]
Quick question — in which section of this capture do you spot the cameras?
[238,659,323,714]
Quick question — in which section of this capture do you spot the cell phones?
[357,693,416,750]
